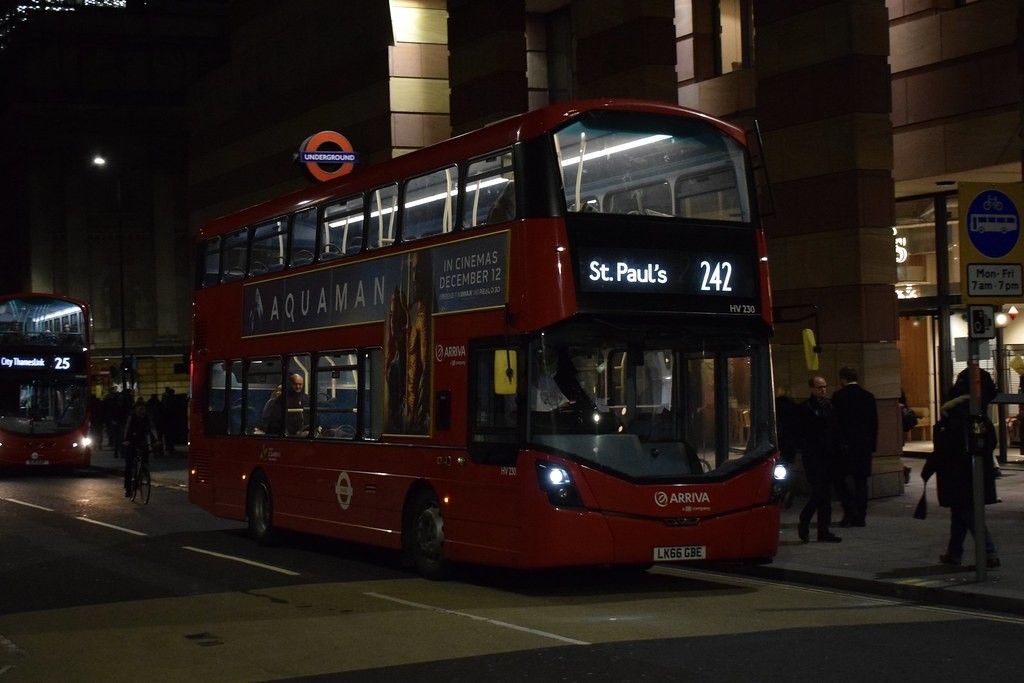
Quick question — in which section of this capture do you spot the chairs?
[65,327,82,344]
[569,204,599,212]
[224,267,246,283]
[249,261,269,278]
[268,256,288,273]
[317,392,331,408]
[228,398,259,434]
[320,243,344,260]
[345,237,370,255]
[292,250,314,266]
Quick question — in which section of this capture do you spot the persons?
[262,373,323,437]
[90,386,186,496]
[774,365,878,542]
[488,183,517,225]
[901,359,1024,566]
[38,322,83,346]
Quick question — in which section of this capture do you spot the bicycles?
[126,443,157,505]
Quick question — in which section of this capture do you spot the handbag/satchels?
[914,481,927,520]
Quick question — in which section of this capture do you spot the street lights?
[92,147,126,400]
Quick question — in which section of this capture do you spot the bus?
[0,293,103,474]
[187,96,824,580]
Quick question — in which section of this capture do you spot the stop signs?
[100,367,110,377]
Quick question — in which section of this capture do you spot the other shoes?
[903,465,912,484]
[817,528,843,543]
[123,490,134,498]
[797,522,810,544]
[982,550,1002,567]
[940,544,964,569]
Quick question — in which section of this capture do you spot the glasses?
[815,385,827,390]
